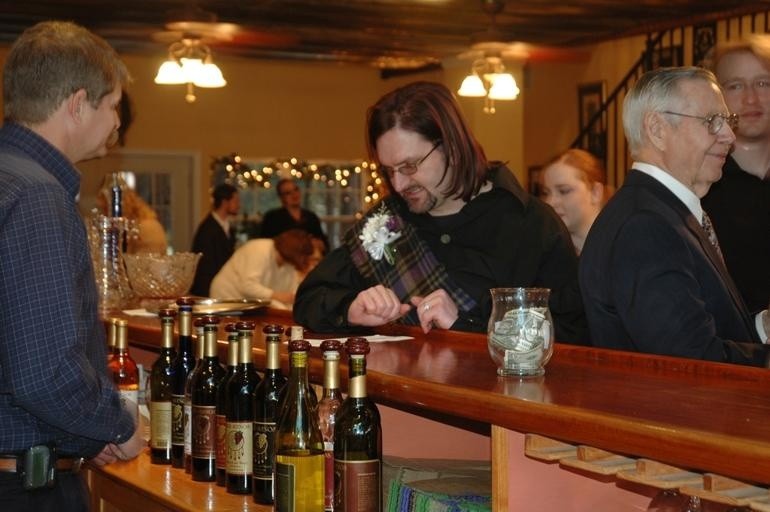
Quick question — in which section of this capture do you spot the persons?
[257,179,331,253]
[536,142,614,260]
[570,61,770,366]
[694,35,770,312]
[289,78,592,346]
[187,182,239,300]
[206,230,316,312]
[2,18,150,511]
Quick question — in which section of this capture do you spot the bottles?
[106,319,139,423]
[486,286,556,377]
[108,183,127,280]
[105,316,121,365]
[148,306,383,511]
[79,215,136,309]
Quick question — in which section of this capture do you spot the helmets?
[166,294,273,316]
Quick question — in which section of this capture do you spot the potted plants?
[529,166,540,197]
[579,83,605,165]
[641,19,718,74]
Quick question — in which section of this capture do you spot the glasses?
[455,50,520,114]
[153,31,227,101]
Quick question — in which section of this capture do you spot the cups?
[659,110,739,135]
[377,142,441,178]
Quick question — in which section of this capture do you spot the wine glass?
[422,300,430,313]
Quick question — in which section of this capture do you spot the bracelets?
[122,250,202,309]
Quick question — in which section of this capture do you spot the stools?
[0,458,86,474]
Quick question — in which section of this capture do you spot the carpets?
[702,210,727,271]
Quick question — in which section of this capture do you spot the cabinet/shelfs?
[106,319,139,423]
[486,286,556,377]
[79,215,136,309]
[108,183,127,280]
[105,316,121,365]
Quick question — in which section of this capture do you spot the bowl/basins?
[122,250,202,309]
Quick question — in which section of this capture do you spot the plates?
[166,294,273,316]
